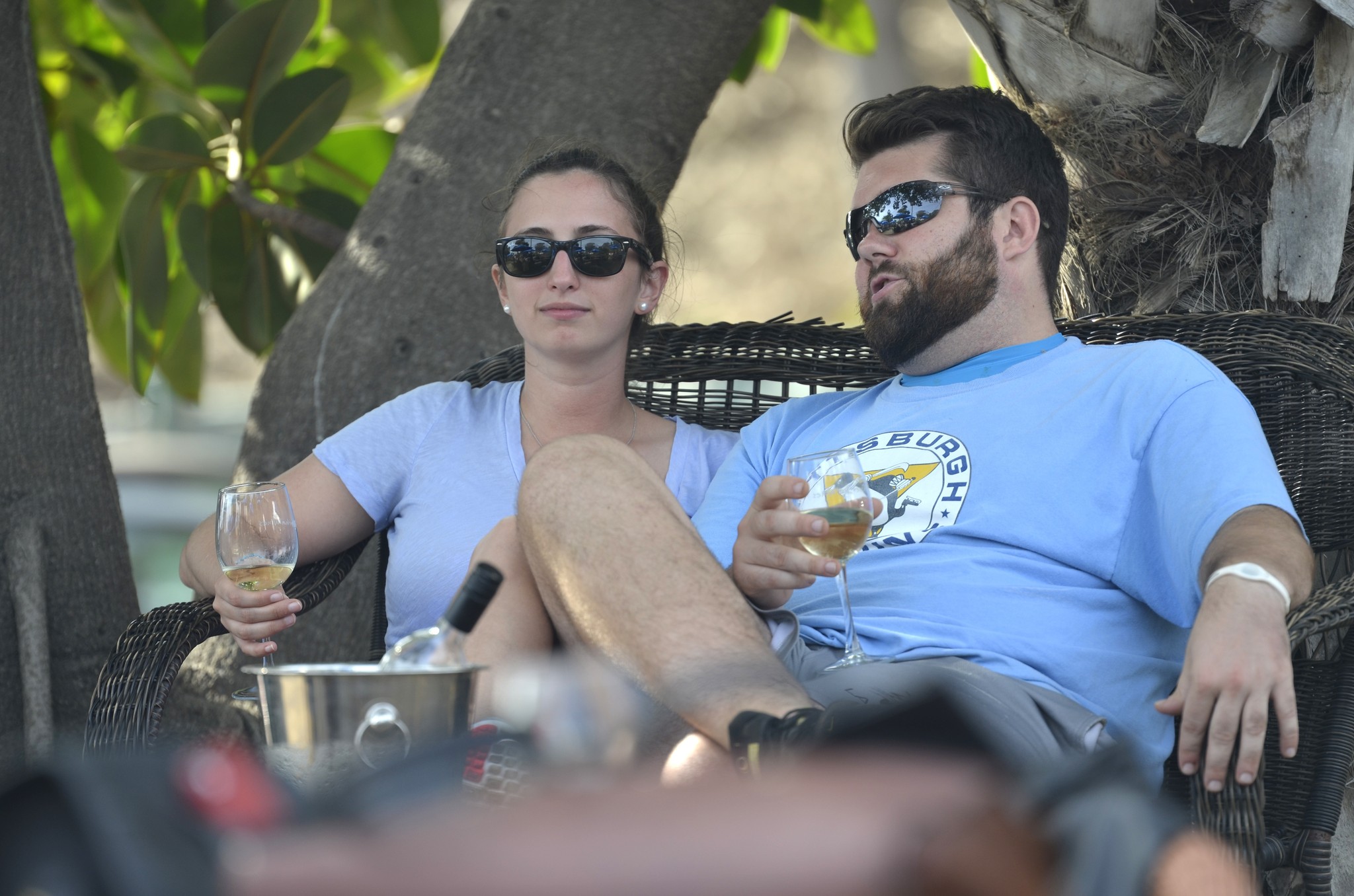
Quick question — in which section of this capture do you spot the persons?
[520,85,1316,896]
[180,151,740,728]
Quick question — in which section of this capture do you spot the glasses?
[495,236,656,278]
[843,179,1049,262]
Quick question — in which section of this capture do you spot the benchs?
[83,311,1354,896]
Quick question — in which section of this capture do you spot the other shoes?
[729,699,853,780]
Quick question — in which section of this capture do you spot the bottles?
[379,561,505,667]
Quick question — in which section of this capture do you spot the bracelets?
[1205,562,1290,609]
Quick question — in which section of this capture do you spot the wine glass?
[788,447,895,671]
[214,481,299,701]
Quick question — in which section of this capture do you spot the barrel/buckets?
[240,662,489,810]
